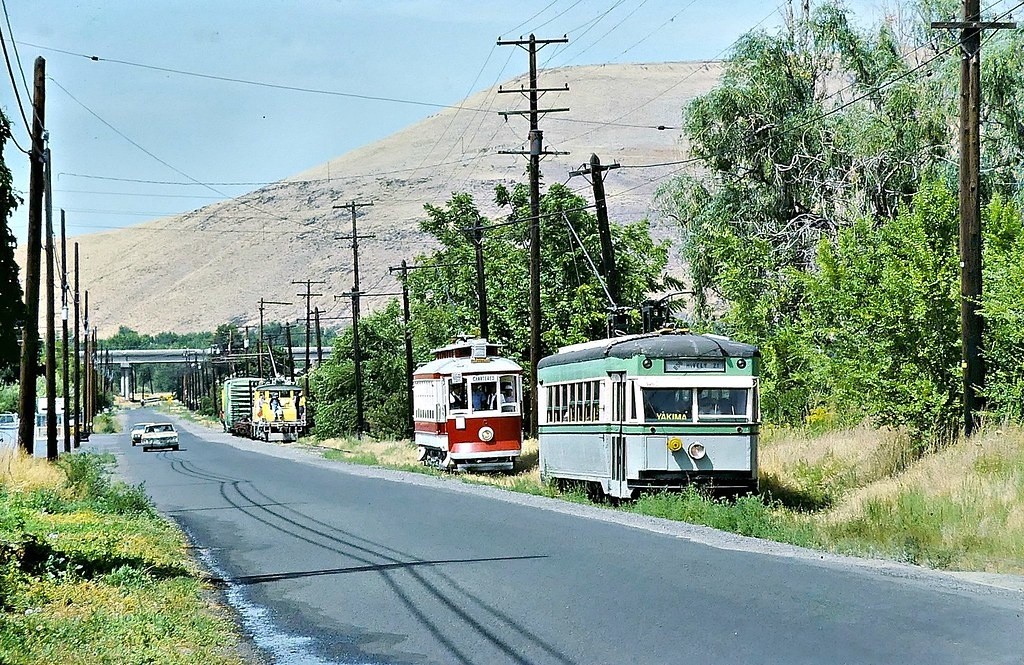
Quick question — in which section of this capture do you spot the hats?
[451,384,462,389]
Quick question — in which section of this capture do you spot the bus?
[535,304,764,505]
[219,372,307,443]
[412,333,522,473]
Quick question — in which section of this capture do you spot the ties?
[489,394,492,404]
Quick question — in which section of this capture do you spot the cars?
[131,422,155,446]
[141,423,179,451]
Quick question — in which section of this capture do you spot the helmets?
[504,385,513,391]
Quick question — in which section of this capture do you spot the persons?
[269,393,284,420]
[257,394,268,419]
[472,385,487,410]
[450,385,464,408]
[220,410,226,431]
[294,392,306,419]
[487,385,514,409]
